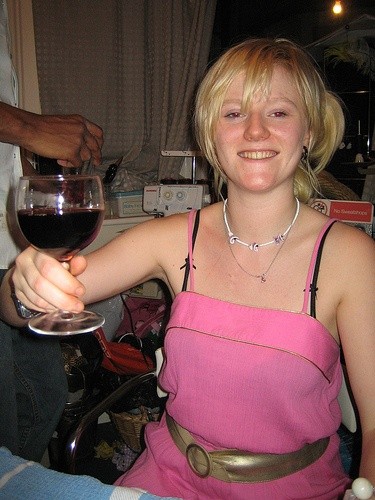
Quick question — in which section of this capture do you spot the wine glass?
[14,173,105,335]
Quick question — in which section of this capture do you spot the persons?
[0,0,104,464]
[0,36,375,500]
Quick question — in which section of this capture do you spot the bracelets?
[11,288,43,320]
[344,477,375,500]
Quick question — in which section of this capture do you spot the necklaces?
[223,197,300,283]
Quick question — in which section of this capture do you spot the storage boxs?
[111,186,151,220]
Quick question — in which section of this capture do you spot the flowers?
[94,436,138,469]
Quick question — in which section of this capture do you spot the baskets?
[106,406,165,452]
